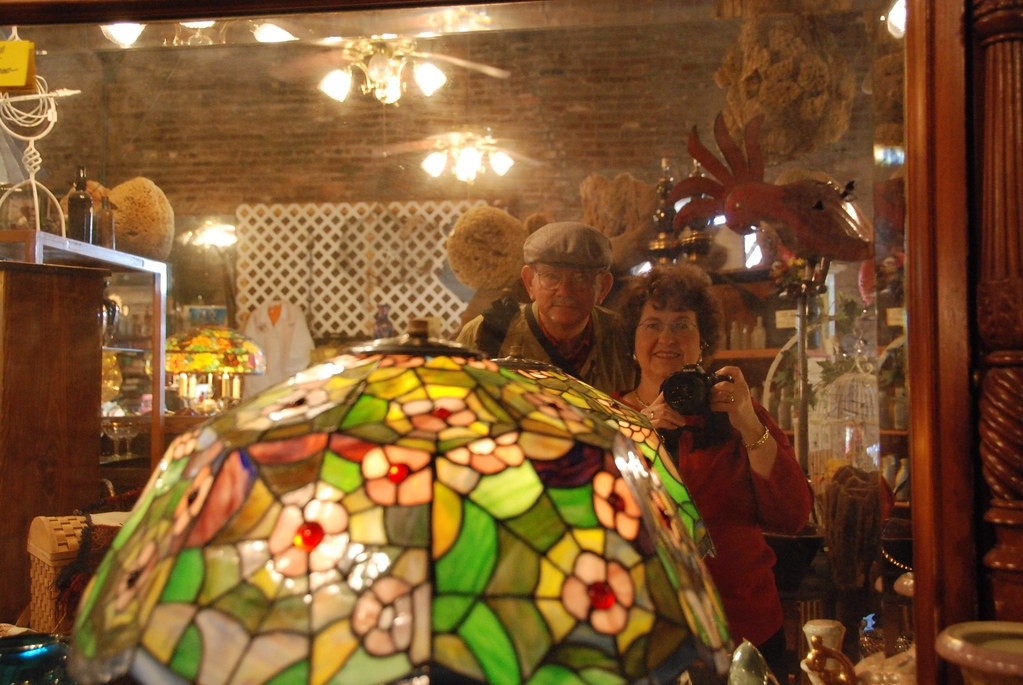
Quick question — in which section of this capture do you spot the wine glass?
[101,423,125,461]
[125,422,140,460]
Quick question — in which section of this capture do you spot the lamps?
[143,322,268,416]
[67,318,735,685]
[192,220,240,328]
[316,52,447,109]
[416,145,513,185]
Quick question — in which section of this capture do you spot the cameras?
[664,363,734,417]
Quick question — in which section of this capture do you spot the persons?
[612,269,814,685]
[457,222,635,396]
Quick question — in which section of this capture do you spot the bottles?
[729,315,793,433]
[68,164,94,245]
[95,197,116,250]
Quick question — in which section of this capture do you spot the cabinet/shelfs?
[709,263,829,365]
[0,258,113,628]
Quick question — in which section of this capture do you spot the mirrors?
[0,0,943,685]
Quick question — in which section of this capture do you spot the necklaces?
[633,391,648,408]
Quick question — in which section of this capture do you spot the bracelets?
[745,426,770,450]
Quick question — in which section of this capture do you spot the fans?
[369,122,545,169]
[282,37,511,84]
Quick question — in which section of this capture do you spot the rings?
[731,393,734,402]
[650,413,654,419]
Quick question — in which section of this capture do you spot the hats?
[524,222,615,270]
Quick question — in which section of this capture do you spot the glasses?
[637,318,700,336]
[531,264,602,290]
